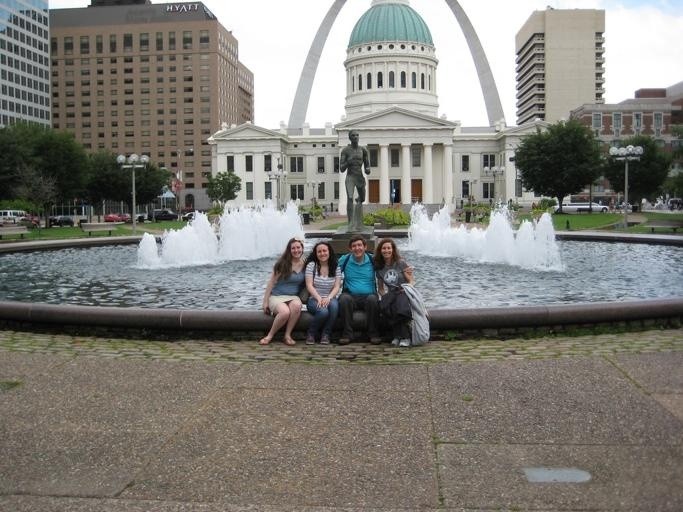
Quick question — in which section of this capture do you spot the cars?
[182,211,209,221]
[105,213,131,222]
[23,217,40,228]
[49,216,74,227]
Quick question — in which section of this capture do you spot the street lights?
[116,151,150,232]
[610,145,644,228]
[483,166,505,207]
[267,170,288,212]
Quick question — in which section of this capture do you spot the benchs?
[152,212,179,222]
[0,224,18,227]
[80,222,118,236]
[641,218,683,232]
[0,226,32,239]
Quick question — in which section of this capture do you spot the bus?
[0,210,27,224]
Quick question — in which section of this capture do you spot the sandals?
[259,337,272,345]
[283,338,296,346]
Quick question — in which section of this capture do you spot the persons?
[370,239,416,348]
[257,237,308,346]
[336,236,412,345]
[299,241,343,345]
[339,128,371,222]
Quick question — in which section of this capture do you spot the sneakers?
[391,338,411,346]
[370,337,382,344]
[305,335,350,345]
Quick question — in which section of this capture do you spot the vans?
[148,208,178,221]
[551,202,609,212]
[668,198,682,209]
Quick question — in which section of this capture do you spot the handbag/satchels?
[380,291,411,328]
[300,286,310,304]
